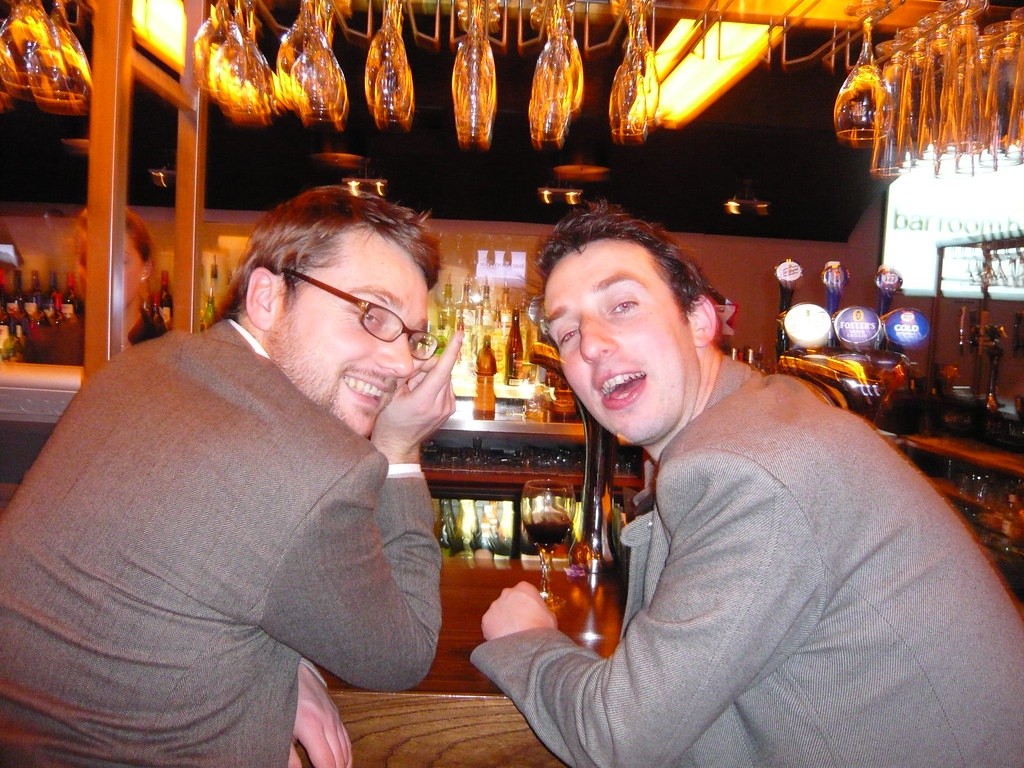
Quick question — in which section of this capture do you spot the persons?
[1,185,465,768]
[471,210,1024,768]
[22,194,167,365]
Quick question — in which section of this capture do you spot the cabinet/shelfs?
[897,432,1024,597]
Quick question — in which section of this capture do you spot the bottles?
[428,275,539,386]
[0,264,219,347]
[472,336,497,421]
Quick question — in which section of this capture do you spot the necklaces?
[127,313,140,333]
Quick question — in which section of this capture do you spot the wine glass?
[835,0,1024,180]
[521,479,576,609]
[0,0,661,142]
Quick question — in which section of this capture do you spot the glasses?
[281,268,440,361]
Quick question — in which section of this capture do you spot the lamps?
[149,162,177,187]
[342,165,388,196]
[724,191,769,215]
[537,174,583,205]
